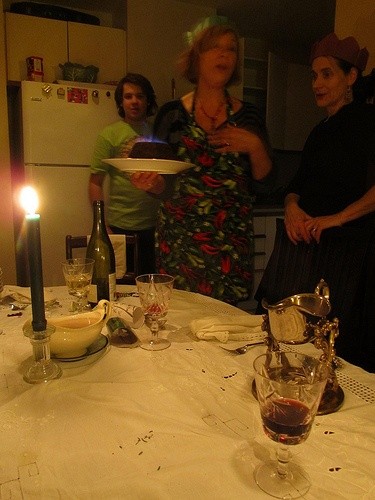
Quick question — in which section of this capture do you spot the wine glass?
[252,350,329,500]
[61,257,95,314]
[136,273,175,351]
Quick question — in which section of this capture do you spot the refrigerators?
[10,81,123,285]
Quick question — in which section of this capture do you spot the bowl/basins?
[59,62,98,83]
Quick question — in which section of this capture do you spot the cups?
[111,301,144,329]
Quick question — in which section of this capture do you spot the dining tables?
[0,284,375,500]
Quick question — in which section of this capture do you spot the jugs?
[46,299,111,358]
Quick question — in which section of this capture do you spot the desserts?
[128,141,175,160]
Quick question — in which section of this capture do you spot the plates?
[101,157,197,174]
[50,333,109,362]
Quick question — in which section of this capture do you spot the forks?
[219,341,266,355]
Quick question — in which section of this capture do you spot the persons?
[88,72,160,277]
[252,31,374,376]
[128,24,274,313]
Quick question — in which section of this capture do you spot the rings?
[225,139,230,146]
[312,227,317,232]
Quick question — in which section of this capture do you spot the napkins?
[190,315,267,343]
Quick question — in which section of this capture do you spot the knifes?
[117,292,138,297]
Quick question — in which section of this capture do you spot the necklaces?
[200,102,223,130]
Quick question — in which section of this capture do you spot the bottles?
[84,198,117,309]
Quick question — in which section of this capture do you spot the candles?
[19,186,48,332]
